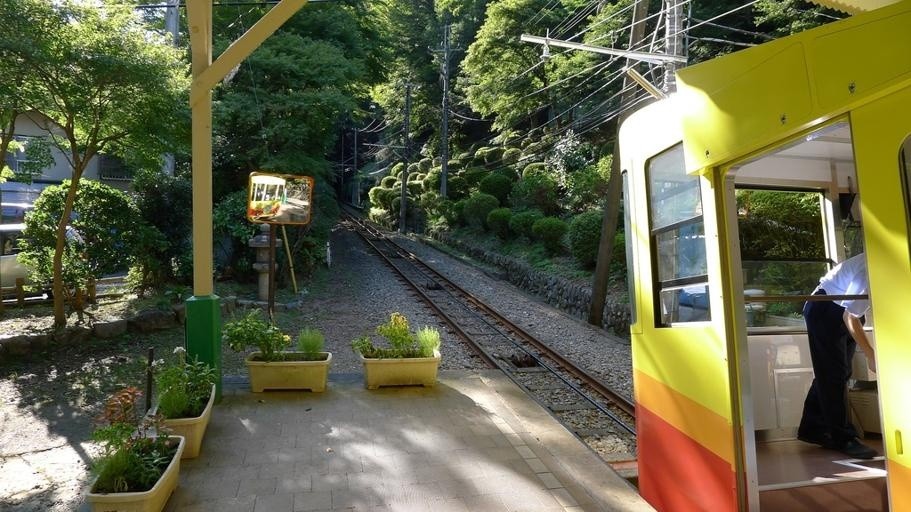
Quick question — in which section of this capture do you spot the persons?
[797,253,879,460]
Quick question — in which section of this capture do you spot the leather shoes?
[797,428,878,459]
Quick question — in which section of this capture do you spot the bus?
[250,175,286,221]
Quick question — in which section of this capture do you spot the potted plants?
[84,344,217,512]
[350,310,441,391]
[220,304,333,394]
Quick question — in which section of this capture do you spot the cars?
[0,199,87,300]
